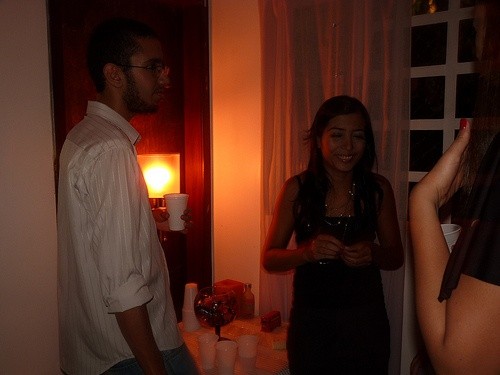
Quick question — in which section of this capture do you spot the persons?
[56,16,202,375]
[262,94,405,375]
[408,0,500,375]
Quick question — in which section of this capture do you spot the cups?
[183,284,199,332]
[164,193,189,231]
[198,334,219,370]
[441,223,462,254]
[238,333,259,369]
[215,340,238,375]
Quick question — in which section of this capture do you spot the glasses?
[122,63,163,77]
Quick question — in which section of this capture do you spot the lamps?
[136,154,181,246]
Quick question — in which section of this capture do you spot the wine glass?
[193,286,237,342]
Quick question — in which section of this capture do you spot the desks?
[170,314,290,375]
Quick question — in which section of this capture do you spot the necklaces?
[316,182,357,264]
